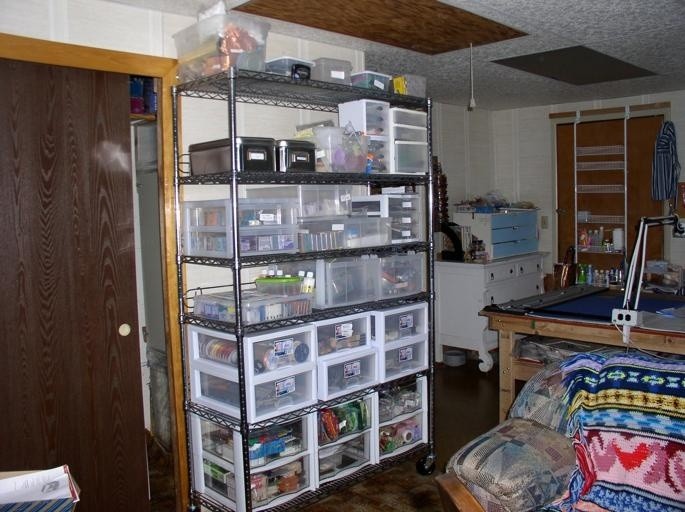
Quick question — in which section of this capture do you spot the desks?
[478,285,684,432]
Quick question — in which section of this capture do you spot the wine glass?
[460,226,473,262]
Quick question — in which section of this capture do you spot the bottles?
[473,239,487,264]
[579,226,624,287]
[256,269,314,294]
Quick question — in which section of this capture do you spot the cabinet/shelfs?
[436,206,550,374]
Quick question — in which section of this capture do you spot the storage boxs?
[171,12,434,511]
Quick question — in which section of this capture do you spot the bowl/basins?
[444,350,466,367]
